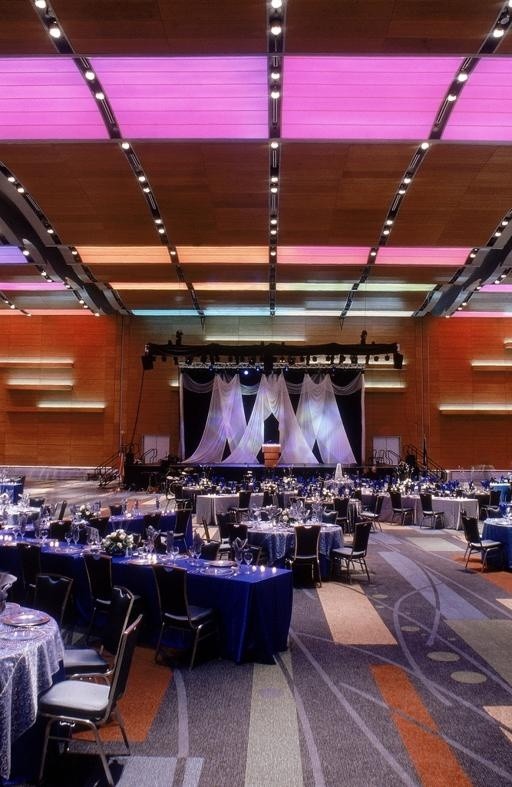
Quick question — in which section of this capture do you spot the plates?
[200,567,234,576]
[208,559,234,567]
[0,627,45,641]
[3,612,49,626]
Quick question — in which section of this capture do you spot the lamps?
[141,331,404,377]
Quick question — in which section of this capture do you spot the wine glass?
[189,546,195,565]
[87,536,94,545]
[170,546,179,566]
[20,531,25,539]
[73,532,80,545]
[244,553,253,574]
[40,531,48,541]
[94,536,102,544]
[234,553,243,567]
[65,533,72,545]
[14,530,19,539]
[194,548,201,565]
